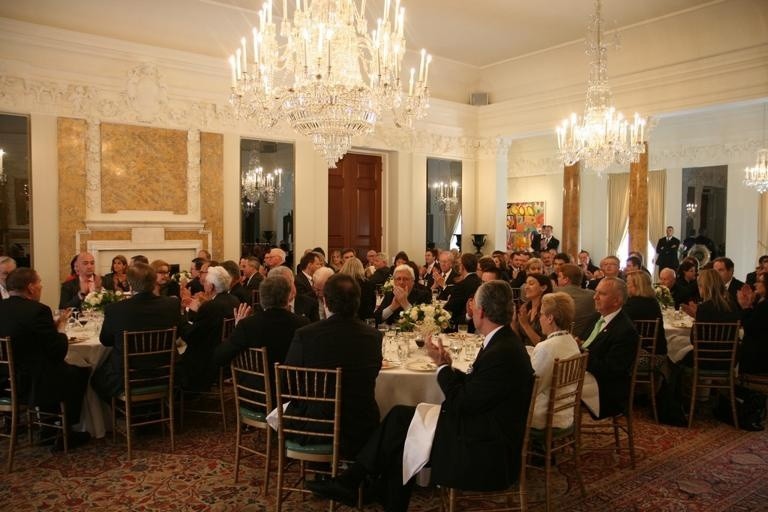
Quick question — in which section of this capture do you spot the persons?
[1,223,768,512]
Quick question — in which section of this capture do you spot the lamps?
[432,182,457,215]
[554,1,649,181]
[741,151,764,191]
[226,0,431,170]
[239,152,282,218]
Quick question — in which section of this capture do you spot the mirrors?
[681,165,728,262]
[240,137,294,268]
[427,157,462,257]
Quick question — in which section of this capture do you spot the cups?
[377,324,476,370]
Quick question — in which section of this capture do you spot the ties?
[582,318,605,348]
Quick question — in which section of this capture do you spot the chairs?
[274,361,363,512]
[688,323,739,429]
[112,327,178,461]
[251,289,262,310]
[631,318,661,423]
[1,335,70,472]
[181,318,233,431]
[533,349,586,509]
[228,347,272,494]
[581,334,641,467]
[443,378,538,509]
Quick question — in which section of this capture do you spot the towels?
[265,400,291,433]
[403,401,441,487]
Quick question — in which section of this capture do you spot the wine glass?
[432,289,438,302]
[68,307,89,335]
